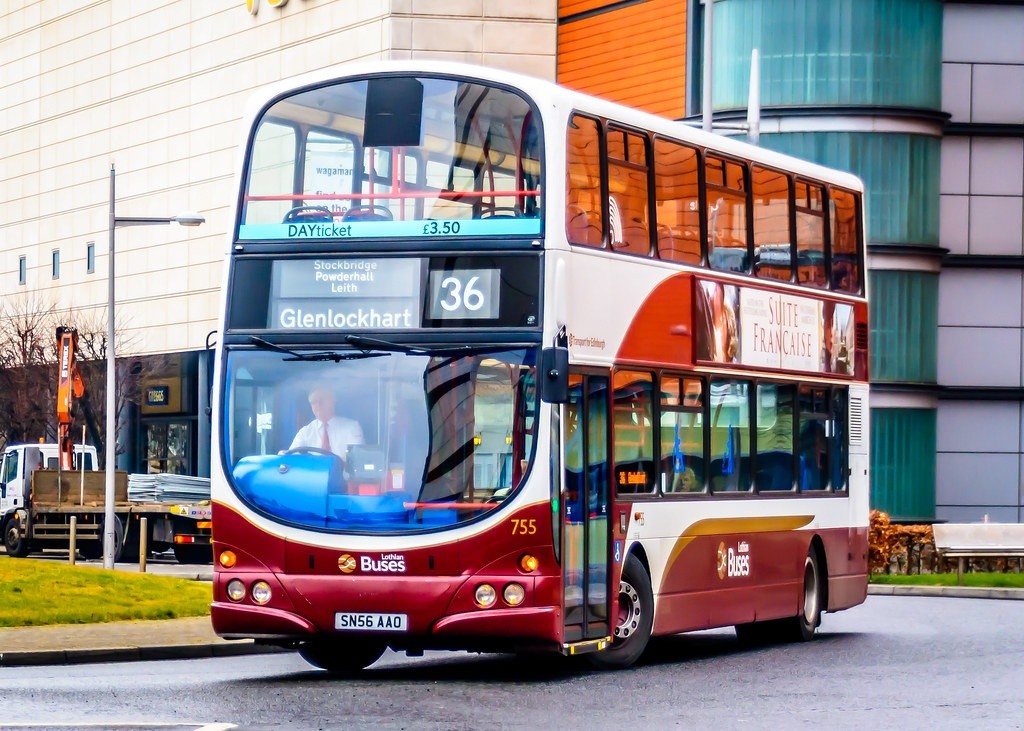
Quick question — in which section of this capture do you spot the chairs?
[473,207,524,219]
[340,205,393,221]
[281,206,334,224]
[565,204,860,294]
[562,452,846,509]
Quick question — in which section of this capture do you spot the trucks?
[0,444,214,565]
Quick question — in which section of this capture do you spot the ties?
[322,421,332,451]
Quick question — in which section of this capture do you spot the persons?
[278,387,365,481]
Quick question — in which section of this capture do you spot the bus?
[205,58,868,673]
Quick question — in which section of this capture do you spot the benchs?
[932,521,1024,586]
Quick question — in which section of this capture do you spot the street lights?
[102,160,207,570]
